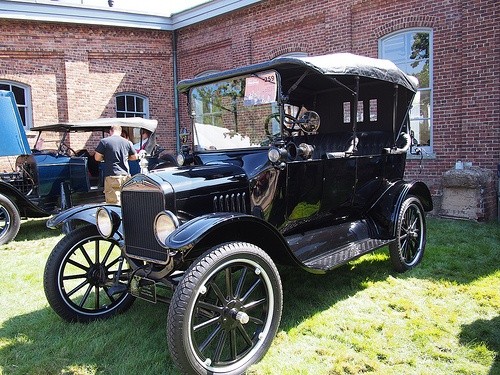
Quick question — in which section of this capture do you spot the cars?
[0,89,164,250]
[39,53,433,375]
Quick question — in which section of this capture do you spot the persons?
[121,129,130,140]
[96,125,139,205]
[133,128,152,154]
